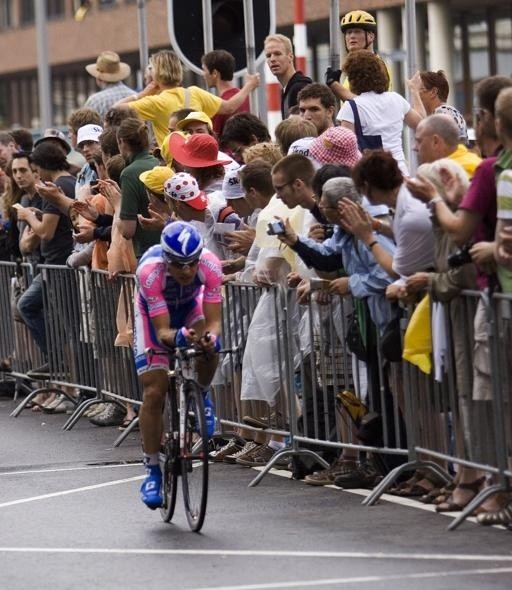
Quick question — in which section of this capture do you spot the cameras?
[319,225,333,239]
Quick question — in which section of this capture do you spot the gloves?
[207,334,221,354]
[324,68,342,87]
[174,327,190,347]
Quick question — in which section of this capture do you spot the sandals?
[418,488,440,503]
[398,473,436,497]
[472,492,511,516]
[369,476,385,490]
[387,471,424,495]
[431,483,457,504]
[477,504,512,526]
[435,476,486,512]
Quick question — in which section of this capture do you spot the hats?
[85,51,130,82]
[163,172,209,210]
[287,137,323,172]
[223,165,246,200]
[160,130,187,168]
[169,132,232,167]
[139,166,176,194]
[77,124,103,148]
[34,129,72,155]
[175,112,213,131]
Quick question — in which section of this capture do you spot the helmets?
[341,10,376,35]
[160,221,203,262]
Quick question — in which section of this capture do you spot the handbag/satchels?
[347,99,383,152]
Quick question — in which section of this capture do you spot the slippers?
[24,401,37,408]
[32,404,44,412]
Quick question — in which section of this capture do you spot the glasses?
[275,183,289,192]
[166,258,199,269]
[317,202,337,213]
[231,144,244,160]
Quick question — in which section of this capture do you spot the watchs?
[365,240,377,252]
[424,196,445,206]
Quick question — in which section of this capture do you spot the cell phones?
[12,203,22,209]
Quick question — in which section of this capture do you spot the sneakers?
[87,403,108,417]
[223,442,257,462]
[90,407,127,426]
[242,412,288,430]
[208,438,229,452]
[65,394,96,414]
[82,404,98,417]
[119,414,138,431]
[272,456,293,471]
[43,395,77,413]
[334,471,378,489]
[1,358,12,372]
[26,362,70,380]
[305,458,361,485]
[235,444,278,466]
[140,464,163,506]
[192,391,216,441]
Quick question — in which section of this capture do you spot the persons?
[66,200,97,417]
[418,69,470,143]
[117,119,161,429]
[265,33,310,117]
[416,157,473,201]
[220,159,304,467]
[114,47,260,152]
[411,112,481,177]
[17,142,77,413]
[0,132,23,261]
[104,153,128,180]
[274,117,319,157]
[126,222,223,508]
[338,148,451,496]
[68,108,103,150]
[71,153,127,426]
[8,129,33,150]
[268,152,333,239]
[202,51,248,133]
[298,82,338,132]
[243,140,283,168]
[12,153,55,413]
[473,87,512,531]
[105,108,138,129]
[99,126,118,162]
[267,175,403,491]
[84,51,137,112]
[338,10,391,105]
[73,123,105,201]
[402,75,512,515]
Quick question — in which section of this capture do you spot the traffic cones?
[265,61,282,142]
[293,0,307,72]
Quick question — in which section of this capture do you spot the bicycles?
[148,330,240,533]
